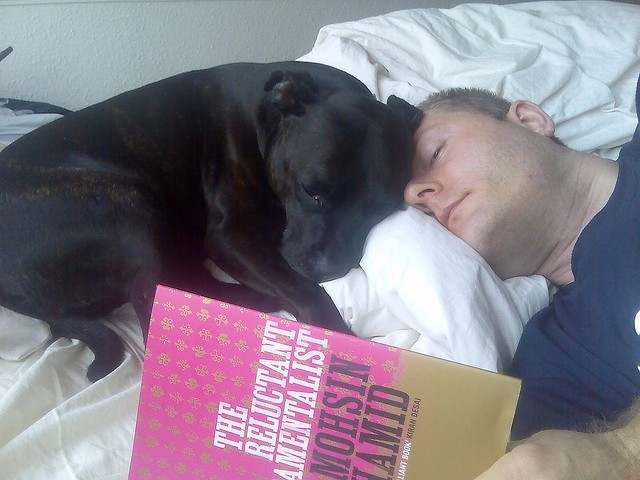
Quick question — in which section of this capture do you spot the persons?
[402,68,640,479]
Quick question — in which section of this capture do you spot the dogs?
[0,60,424,384]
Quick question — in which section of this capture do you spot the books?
[125,281,524,479]
